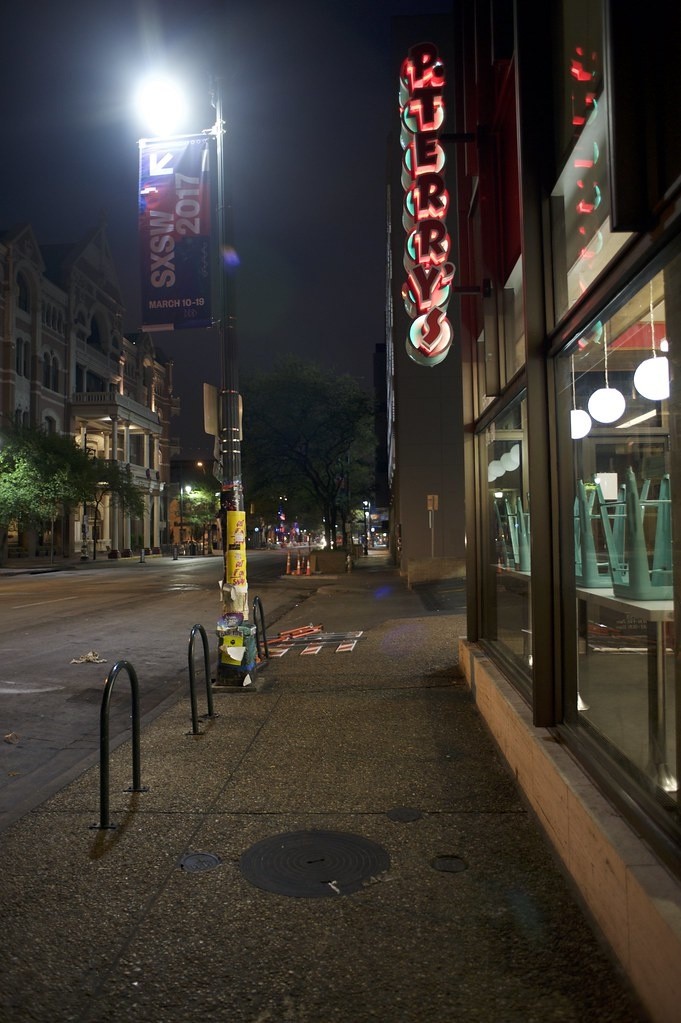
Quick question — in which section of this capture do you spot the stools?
[493,465,673,601]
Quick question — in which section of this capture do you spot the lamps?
[570,355,591,440]
[588,323,626,423]
[488,443,519,483]
[634,280,670,401]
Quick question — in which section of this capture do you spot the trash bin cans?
[140,549,145,563]
[173,548,178,560]
[214,622,258,686]
[189,545,196,555]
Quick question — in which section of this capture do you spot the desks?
[490,563,678,793]
